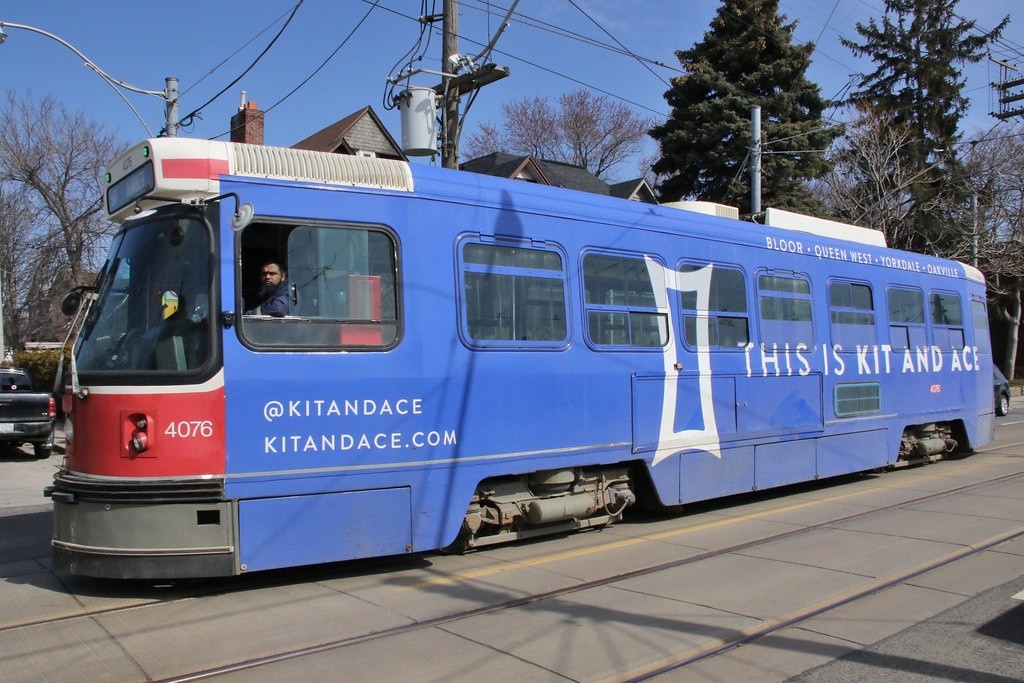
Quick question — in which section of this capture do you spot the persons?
[244,261,304,319]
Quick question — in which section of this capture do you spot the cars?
[993,363,1011,416]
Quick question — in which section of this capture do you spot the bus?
[55,139,996,581]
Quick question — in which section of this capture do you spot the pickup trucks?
[0,367,56,459]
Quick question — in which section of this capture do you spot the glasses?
[262,271,280,276]
